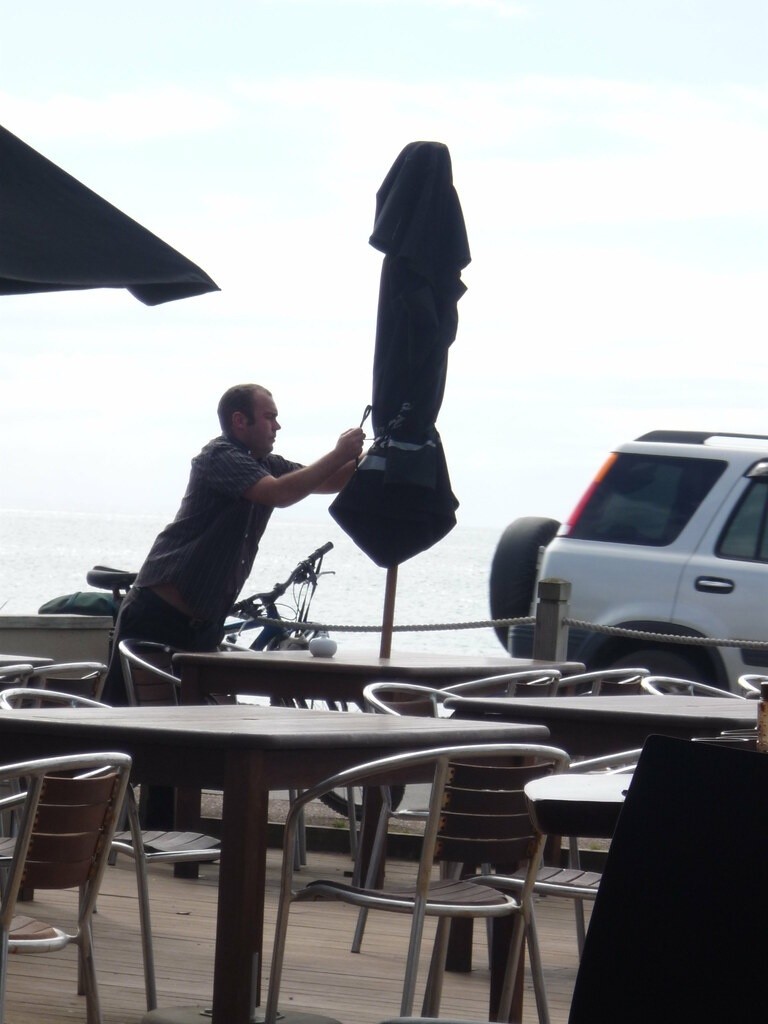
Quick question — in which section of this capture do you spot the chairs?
[0,638,768,1024]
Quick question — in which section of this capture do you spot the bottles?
[757,681,768,752]
[222,633,237,651]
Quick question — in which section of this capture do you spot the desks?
[172,649,582,897]
[445,696,768,971]
[0,703,550,1024]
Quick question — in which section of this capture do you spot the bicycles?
[87,546,407,817]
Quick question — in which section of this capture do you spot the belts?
[137,588,213,624]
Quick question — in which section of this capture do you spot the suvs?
[487,431,767,751]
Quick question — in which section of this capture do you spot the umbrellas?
[327,140,473,658]
[0,123,221,308]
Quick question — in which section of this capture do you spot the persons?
[99,386,367,854]
